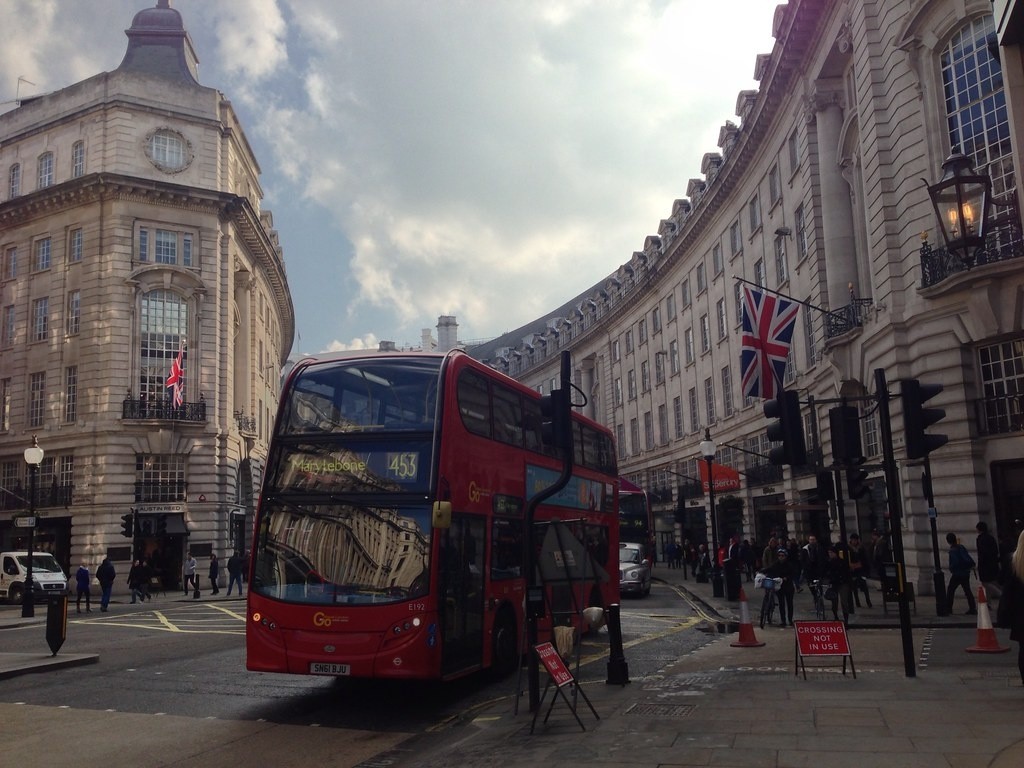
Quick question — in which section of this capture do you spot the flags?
[166,348,184,409]
[741,286,800,400]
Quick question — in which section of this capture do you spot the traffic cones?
[729,588,766,647]
[965,586,1012,653]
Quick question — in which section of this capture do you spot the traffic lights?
[763,390,806,463]
[900,380,949,467]
[843,455,869,500]
[120,514,133,538]
[156,514,168,536]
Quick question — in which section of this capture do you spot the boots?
[77,604,82,613]
[85,606,92,612]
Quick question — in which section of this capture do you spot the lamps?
[656,350,667,355]
[918,143,1021,272]
[775,226,791,235]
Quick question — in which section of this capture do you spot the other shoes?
[789,621,794,626]
[948,608,953,613]
[777,621,786,626]
[867,602,873,608]
[966,608,977,615]
[211,590,219,596]
[844,613,849,621]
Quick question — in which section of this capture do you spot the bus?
[245,350,620,684]
[619,489,657,570]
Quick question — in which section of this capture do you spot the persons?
[227,550,242,596]
[756,548,795,627]
[208,554,220,596]
[76,561,92,613]
[183,552,197,595]
[821,546,850,620]
[666,538,709,577]
[975,522,1002,608]
[997,529,1024,683]
[127,559,152,604]
[717,535,828,600]
[96,556,115,612]
[848,528,891,608]
[946,532,978,615]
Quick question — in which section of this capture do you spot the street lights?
[21,447,44,617]
[699,441,724,599]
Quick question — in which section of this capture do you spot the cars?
[0,551,68,606]
[620,541,652,596]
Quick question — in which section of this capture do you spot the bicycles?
[811,580,826,620]
[757,577,780,629]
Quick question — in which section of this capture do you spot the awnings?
[137,515,190,537]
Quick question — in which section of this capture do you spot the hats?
[777,548,788,556]
[946,533,956,544]
[977,522,988,532]
[872,528,881,536]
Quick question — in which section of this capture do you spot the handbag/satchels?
[825,584,837,601]
[960,546,978,580]
[850,562,861,570]
[695,573,709,583]
